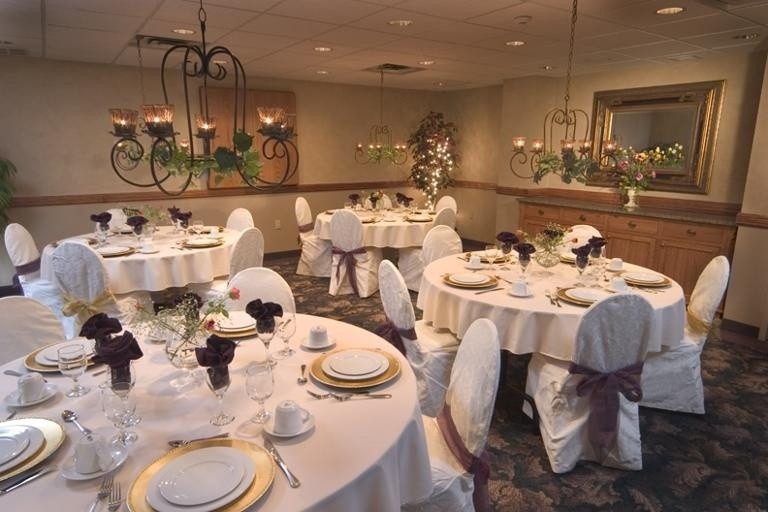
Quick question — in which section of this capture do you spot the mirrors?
[584,78,724,195]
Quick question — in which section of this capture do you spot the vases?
[625,186,639,209]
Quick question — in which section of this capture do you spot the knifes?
[259,435,301,488]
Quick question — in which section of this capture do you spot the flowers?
[612,142,656,190]
[651,143,686,168]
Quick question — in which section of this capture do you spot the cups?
[467,255,625,296]
[75,436,113,474]
[310,326,327,346]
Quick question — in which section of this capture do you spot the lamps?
[107,1,300,197]
[510,0,620,180]
[353,63,428,165]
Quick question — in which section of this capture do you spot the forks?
[107,481,123,512]
[89,473,117,511]
[306,388,393,401]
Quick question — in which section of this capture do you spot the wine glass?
[93,219,205,250]
[104,364,136,408]
[247,364,276,425]
[101,383,142,443]
[255,321,277,359]
[277,315,296,356]
[57,346,91,398]
[58,313,296,445]
[205,368,235,426]
[485,243,607,286]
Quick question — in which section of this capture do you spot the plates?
[443,251,671,306]
[212,323,257,340]
[124,435,276,511]
[326,208,433,223]
[23,337,97,372]
[301,337,336,350]
[2,417,64,489]
[56,435,129,482]
[52,225,223,257]
[307,346,400,391]
